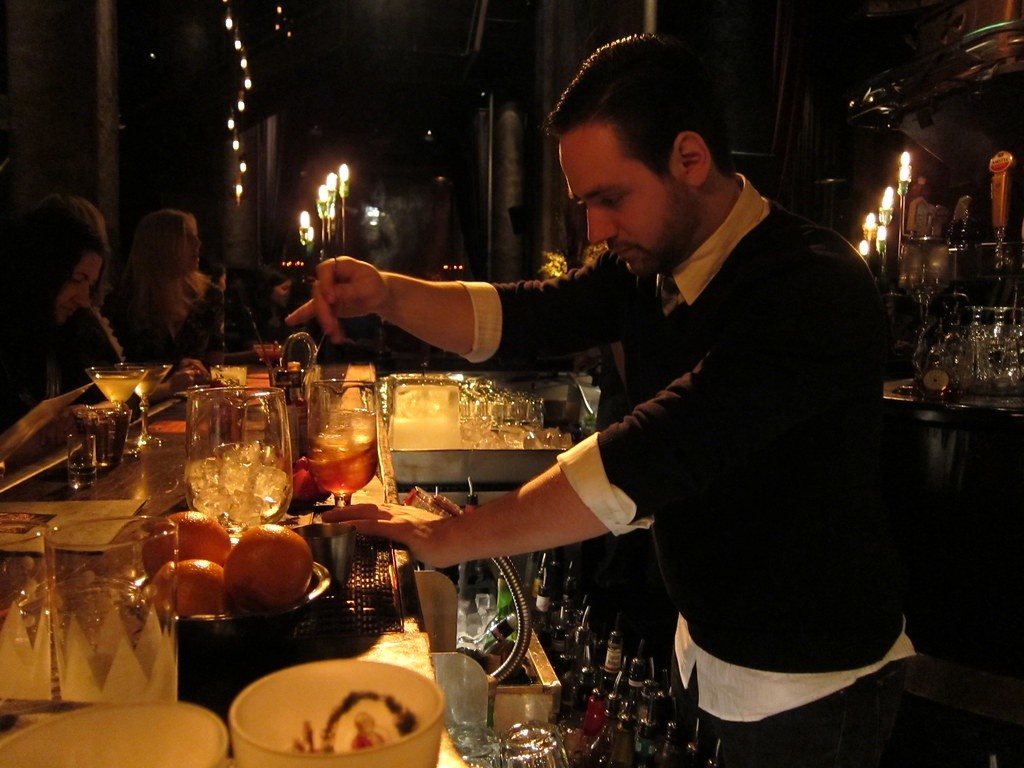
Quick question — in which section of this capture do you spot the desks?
[0,363,380,634]
[883,377,1024,489]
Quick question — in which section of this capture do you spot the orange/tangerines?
[142,511,232,578]
[148,559,225,617]
[223,524,312,610]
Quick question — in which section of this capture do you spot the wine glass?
[913,307,1024,404]
[308,380,379,507]
[85,362,173,461]
[184,387,293,543]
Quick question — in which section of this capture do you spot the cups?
[0,512,179,711]
[292,524,357,601]
[210,366,248,387]
[447,721,502,768]
[502,720,571,768]
[0,701,229,768]
[71,404,132,469]
[67,432,97,489]
[229,659,444,768]
[459,377,544,450]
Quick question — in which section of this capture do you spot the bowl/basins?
[254,345,282,360]
[179,561,332,686]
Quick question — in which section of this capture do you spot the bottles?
[628,639,722,768]
[276,362,301,405]
[585,613,631,768]
[498,550,579,638]
[455,612,518,669]
[552,594,594,728]
[457,495,498,612]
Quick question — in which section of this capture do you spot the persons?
[284,30,916,768]
[0,200,349,472]
